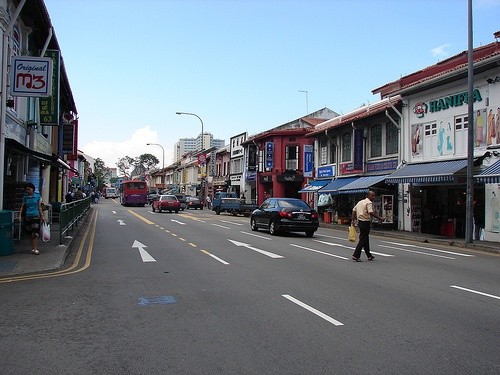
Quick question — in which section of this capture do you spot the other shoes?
[352,256,363,262]
[34,249,40,255]
[31,249,35,253]
[368,256,375,260]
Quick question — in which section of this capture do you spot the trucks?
[211,192,259,217]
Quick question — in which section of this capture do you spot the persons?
[206,193,211,209]
[64,190,99,206]
[437,120,453,154]
[18,183,47,255]
[412,124,423,157]
[487,107,500,144]
[348,189,385,261]
[474,110,483,146]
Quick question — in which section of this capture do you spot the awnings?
[6,137,78,175]
[298,159,500,194]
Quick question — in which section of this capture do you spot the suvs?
[169,193,187,211]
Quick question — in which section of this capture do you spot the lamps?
[493,151,499,156]
[483,151,492,158]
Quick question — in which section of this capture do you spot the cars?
[151,194,182,214]
[185,196,203,210]
[250,198,320,238]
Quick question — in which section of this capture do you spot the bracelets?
[380,216,381,220]
[41,215,43,216]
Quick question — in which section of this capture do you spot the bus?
[118,179,149,207]
[104,187,116,199]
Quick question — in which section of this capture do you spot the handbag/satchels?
[348,223,356,242]
[21,196,26,217]
[42,222,51,242]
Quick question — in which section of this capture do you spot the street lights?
[176,111,204,203]
[147,143,165,195]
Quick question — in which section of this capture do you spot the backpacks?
[66,192,72,203]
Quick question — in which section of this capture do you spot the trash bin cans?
[0,209,14,256]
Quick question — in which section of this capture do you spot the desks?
[45,204,53,225]
